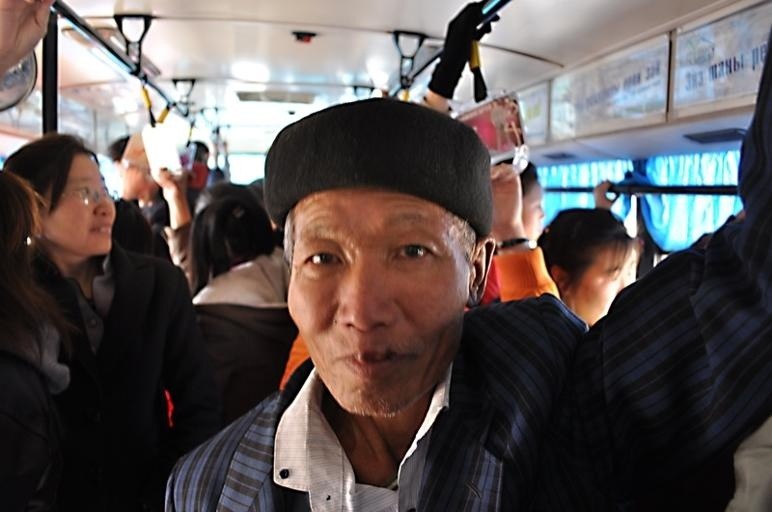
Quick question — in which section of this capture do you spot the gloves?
[427,3,499,98]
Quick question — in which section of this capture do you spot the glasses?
[57,186,118,207]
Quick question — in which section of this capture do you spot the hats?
[264,98,492,234]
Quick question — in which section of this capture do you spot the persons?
[167,29,771,511]
[1,1,312,511]
[419,0,636,318]
